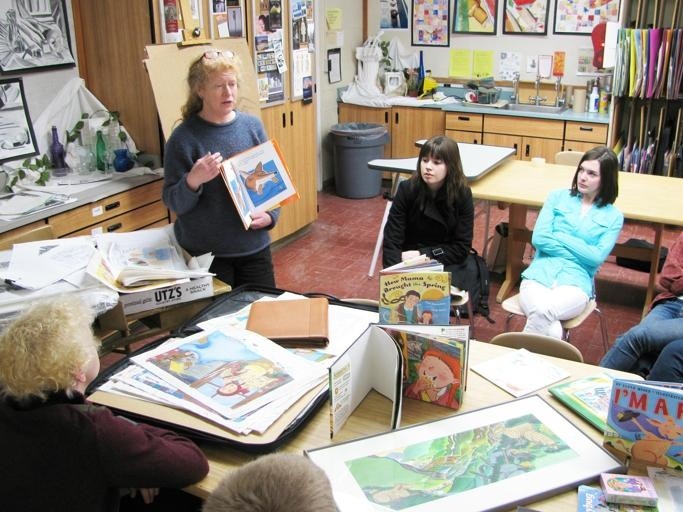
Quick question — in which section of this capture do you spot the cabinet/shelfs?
[444,111,482,145]
[336,92,444,187]
[0,139,172,242]
[71,0,247,223]
[564,121,607,152]
[481,113,565,163]
[245,0,317,253]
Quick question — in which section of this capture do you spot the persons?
[381,136,474,281]
[598,220,682,381]
[156,49,287,291]
[201,453,343,512]
[258,15,274,35]
[520,149,627,342]
[0,290,214,512]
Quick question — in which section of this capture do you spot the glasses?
[191,50,237,66]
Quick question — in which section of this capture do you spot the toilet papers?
[572,87,587,113]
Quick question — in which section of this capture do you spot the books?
[601,379,682,474]
[546,371,613,439]
[211,133,304,236]
[324,320,472,440]
[376,250,457,327]
[243,298,331,347]
[575,483,659,511]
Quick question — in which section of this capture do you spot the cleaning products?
[589,76,599,112]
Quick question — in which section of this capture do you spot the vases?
[111,149,133,173]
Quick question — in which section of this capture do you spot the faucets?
[534,73,542,104]
[554,80,560,107]
[513,70,521,103]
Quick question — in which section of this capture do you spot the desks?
[366,139,517,280]
[464,158,681,327]
[76,284,682,512]
[0,227,233,361]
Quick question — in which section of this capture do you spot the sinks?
[497,103,568,115]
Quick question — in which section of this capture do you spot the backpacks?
[438,249,492,316]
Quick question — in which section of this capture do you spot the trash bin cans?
[331,122,388,199]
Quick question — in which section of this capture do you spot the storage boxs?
[112,245,215,314]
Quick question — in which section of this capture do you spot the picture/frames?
[501,0,550,35]
[451,0,498,35]
[552,0,621,36]
[303,393,630,512]
[410,0,449,46]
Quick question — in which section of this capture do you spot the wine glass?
[99,150,112,177]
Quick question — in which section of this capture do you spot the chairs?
[554,150,586,168]
[488,331,583,361]
[499,266,610,354]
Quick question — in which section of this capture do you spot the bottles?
[95,131,107,171]
[49,128,66,176]
[425,70,431,78]
[416,51,424,94]
[598,90,608,114]
[588,85,599,112]
